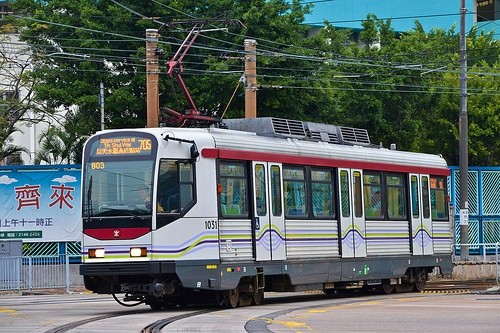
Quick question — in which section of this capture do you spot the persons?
[130,184,163,212]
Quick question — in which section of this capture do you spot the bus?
[79,117,454,308]
